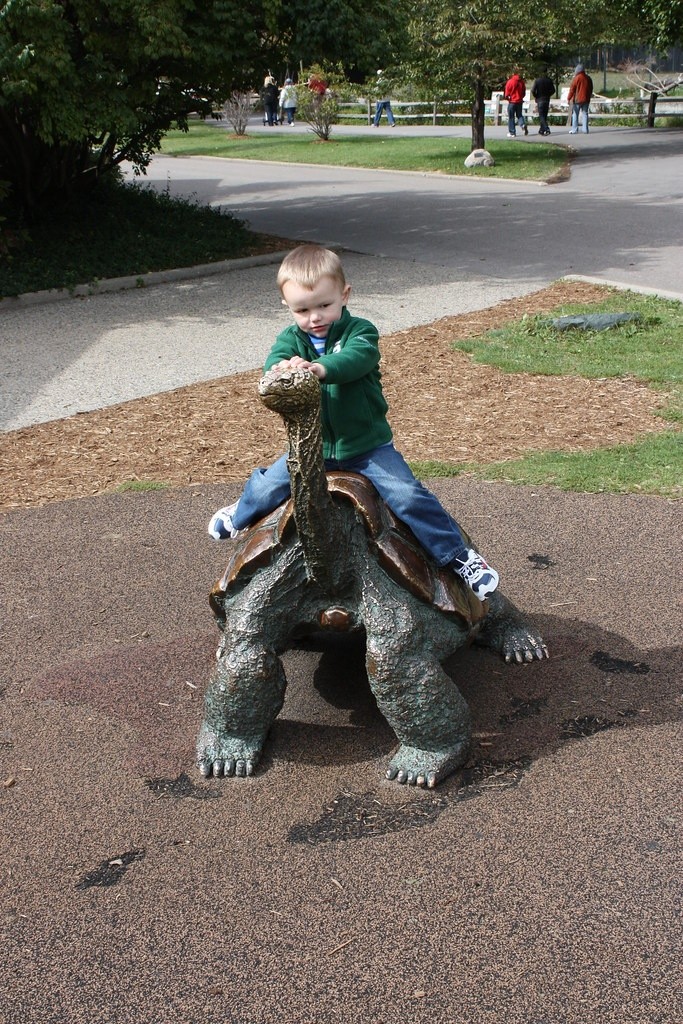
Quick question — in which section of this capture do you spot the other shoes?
[289,122,295,127]
[273,120,278,125]
[390,124,395,127]
[539,130,550,135]
[269,124,273,126]
[372,124,379,128]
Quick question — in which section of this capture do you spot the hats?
[286,79,291,82]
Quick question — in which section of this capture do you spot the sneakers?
[208,503,250,540]
[449,547,500,602]
[569,130,577,134]
[584,132,588,134]
[525,131,528,135]
[507,133,516,137]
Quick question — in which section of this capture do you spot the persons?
[205,245,500,604]
[567,63,593,134]
[531,65,556,136]
[372,69,396,128]
[308,63,330,129]
[260,76,299,127]
[504,66,528,138]
